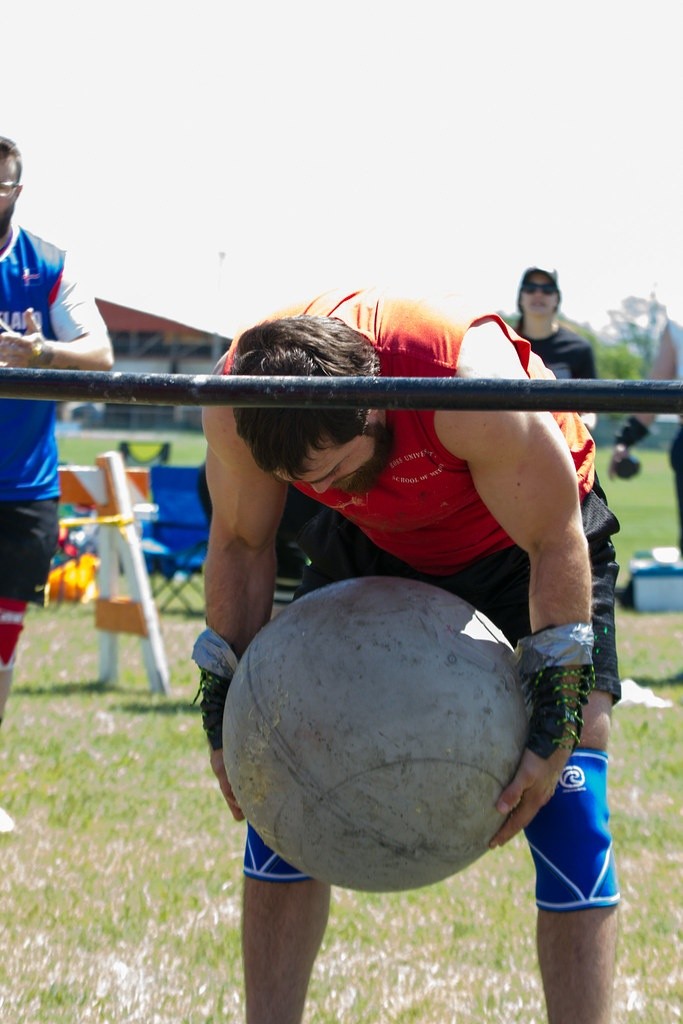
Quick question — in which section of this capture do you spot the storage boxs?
[629,551,683,614]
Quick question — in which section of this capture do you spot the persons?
[512,268,599,434]
[0,135,118,841]
[199,276,627,1023]
[607,311,683,559]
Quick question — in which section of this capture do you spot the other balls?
[223,579,535,893]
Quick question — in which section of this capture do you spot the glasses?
[0,181,20,197]
[522,283,557,295]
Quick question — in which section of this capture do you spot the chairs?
[141,465,211,615]
